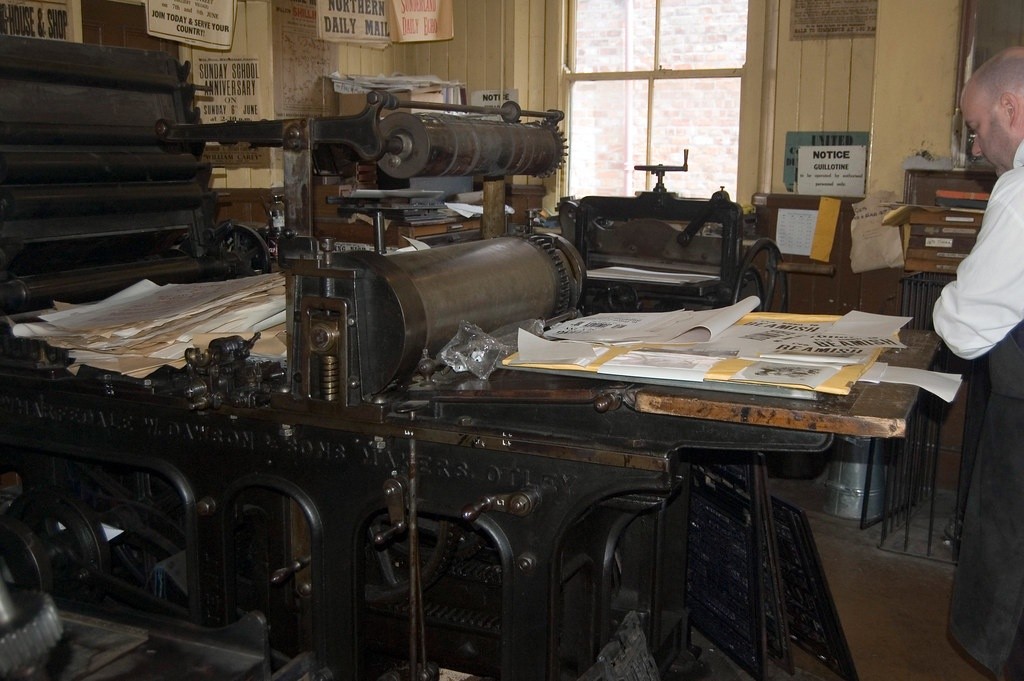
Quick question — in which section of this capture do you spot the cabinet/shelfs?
[314,217,483,249]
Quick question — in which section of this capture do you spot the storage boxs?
[339,85,444,116]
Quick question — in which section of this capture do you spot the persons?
[932,47,1024,681]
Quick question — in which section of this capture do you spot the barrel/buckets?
[823,435,886,520]
